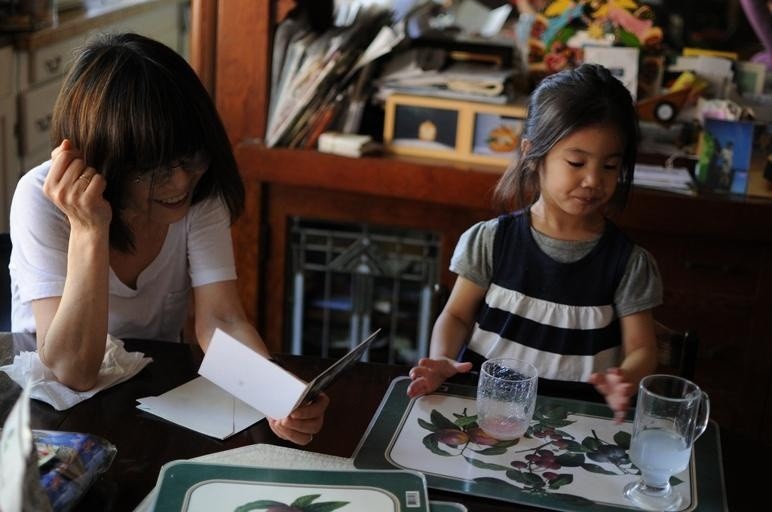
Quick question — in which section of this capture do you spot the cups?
[475,357,540,441]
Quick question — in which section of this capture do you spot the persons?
[4,27,332,453]
[718,139,737,190]
[404,58,668,432]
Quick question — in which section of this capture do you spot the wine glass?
[621,372,711,512]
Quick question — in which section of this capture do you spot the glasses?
[128,151,210,187]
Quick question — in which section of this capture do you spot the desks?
[0,332,771,512]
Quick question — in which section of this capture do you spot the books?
[263,6,523,153]
[627,160,697,192]
